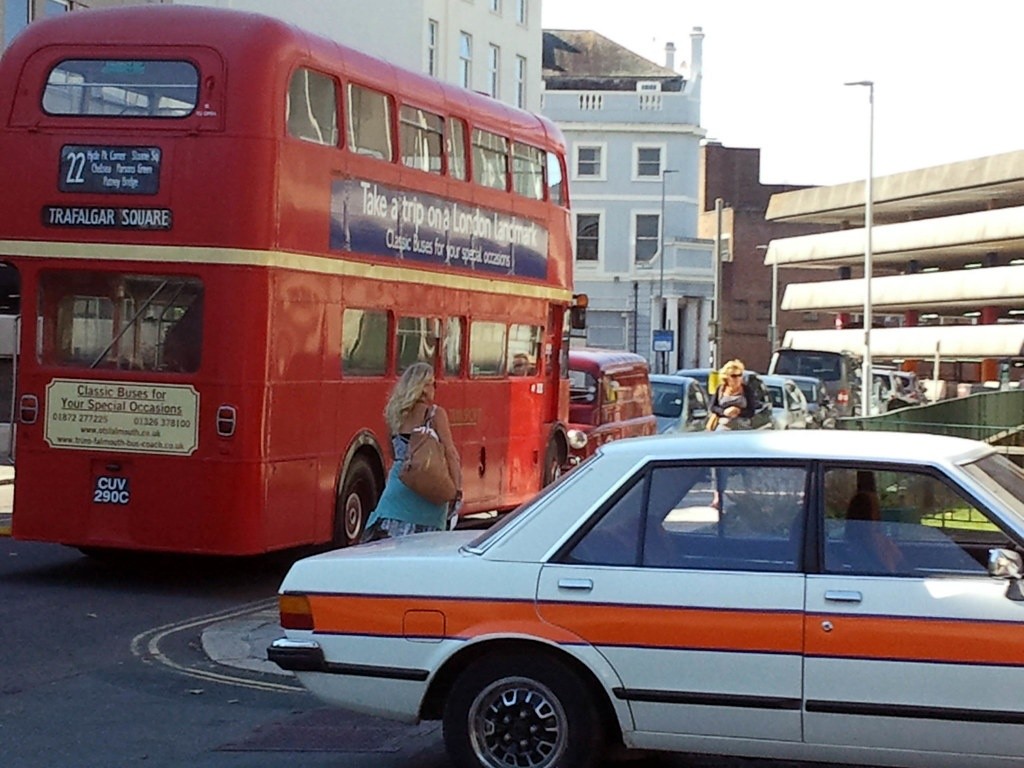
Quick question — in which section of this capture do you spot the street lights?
[660,169,682,373]
[756,245,779,355]
[846,81,876,419]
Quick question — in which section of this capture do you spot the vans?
[767,346,861,419]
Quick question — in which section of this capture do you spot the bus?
[0,3,590,586]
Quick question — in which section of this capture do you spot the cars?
[894,369,930,405]
[647,374,712,436]
[757,374,809,428]
[264,431,1023,768]
[561,347,657,474]
[853,364,895,411]
[785,373,840,429]
[673,367,773,430]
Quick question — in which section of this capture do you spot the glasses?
[730,373,742,378]
[423,381,437,390]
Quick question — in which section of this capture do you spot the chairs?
[571,489,907,577]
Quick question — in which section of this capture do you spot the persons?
[365,363,460,537]
[513,354,528,376]
[709,359,755,431]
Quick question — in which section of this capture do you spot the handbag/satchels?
[704,412,720,432]
[397,403,458,506]
